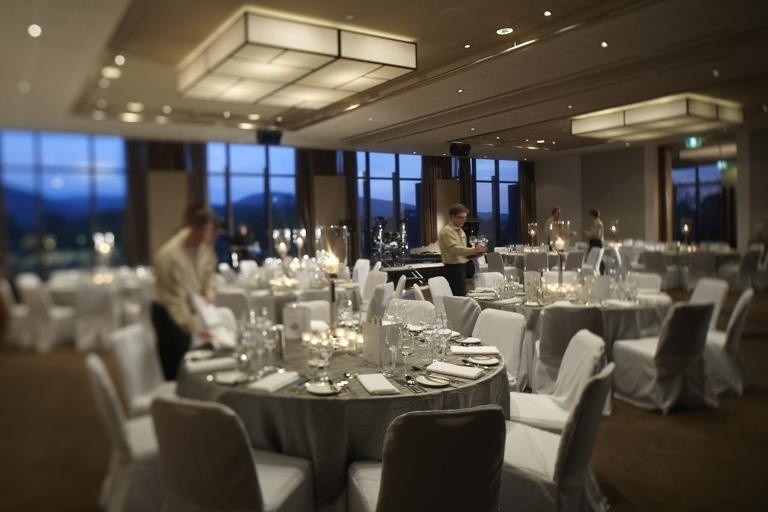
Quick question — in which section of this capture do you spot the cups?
[522,269,543,307]
[305,348,318,379]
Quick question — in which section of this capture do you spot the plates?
[417,374,450,388]
[214,369,249,386]
[454,336,482,344]
[304,379,342,395]
[467,354,499,366]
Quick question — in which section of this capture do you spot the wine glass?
[272,226,308,280]
[545,219,572,297]
[236,306,276,378]
[527,222,539,248]
[385,302,411,336]
[346,310,363,352]
[319,338,334,381]
[607,217,619,241]
[576,264,638,305]
[494,274,520,299]
[680,216,692,246]
[421,316,452,364]
[384,325,414,378]
[313,225,350,355]
[469,237,490,249]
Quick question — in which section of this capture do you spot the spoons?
[404,374,427,394]
[341,376,356,398]
[461,359,496,371]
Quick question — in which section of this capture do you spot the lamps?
[172,11,419,111]
[572,93,744,146]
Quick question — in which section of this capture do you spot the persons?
[544,206,576,252]
[439,203,487,297]
[153,210,216,382]
[582,209,606,276]
